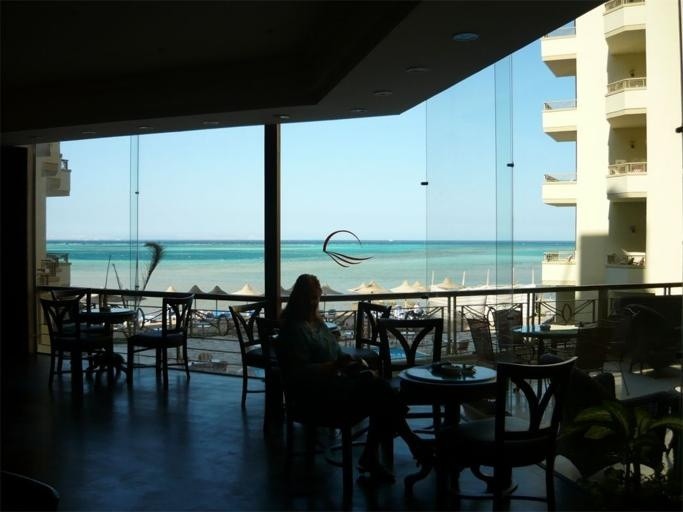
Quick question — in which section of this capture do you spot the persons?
[273,274,435,481]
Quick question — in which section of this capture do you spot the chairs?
[609,248,646,267]
[610,160,647,175]
[0,290,682,512]
